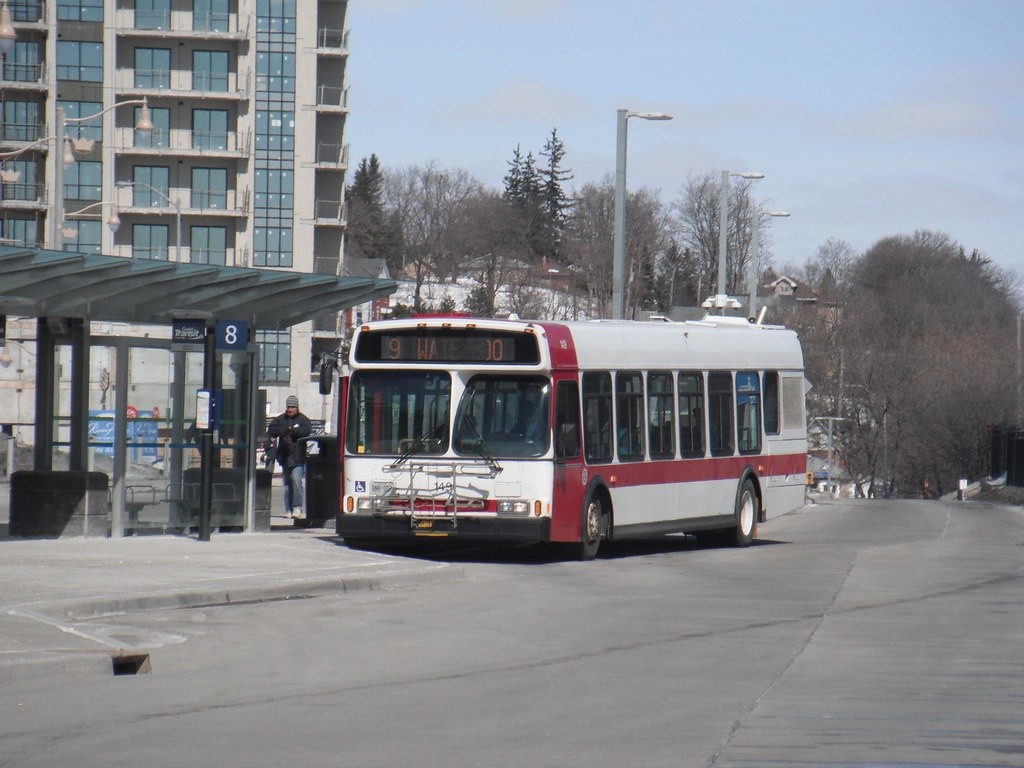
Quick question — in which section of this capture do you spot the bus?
[319,293,808,561]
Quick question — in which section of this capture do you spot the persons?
[264,438,277,477]
[268,395,312,518]
[505,402,547,447]
[459,415,478,437]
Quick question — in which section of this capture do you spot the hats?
[286,396,299,406]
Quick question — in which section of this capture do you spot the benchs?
[160,478,241,537]
[108,485,160,536]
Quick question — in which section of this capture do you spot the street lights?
[716,170,765,295]
[749,209,792,318]
[612,109,674,319]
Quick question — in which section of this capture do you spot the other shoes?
[287,509,301,519]
